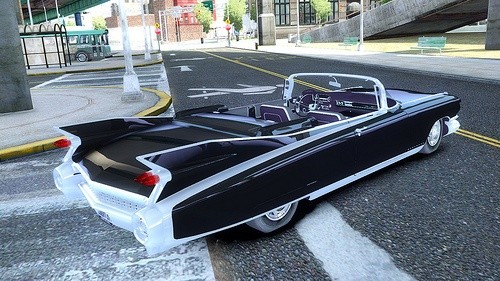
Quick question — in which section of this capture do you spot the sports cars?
[52,70,461,255]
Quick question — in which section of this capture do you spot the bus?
[64,29,114,62]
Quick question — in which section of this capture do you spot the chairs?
[306,111,341,125]
[260,104,292,123]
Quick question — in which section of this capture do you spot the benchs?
[338,37,358,49]
[410,36,448,54]
[299,37,310,48]
